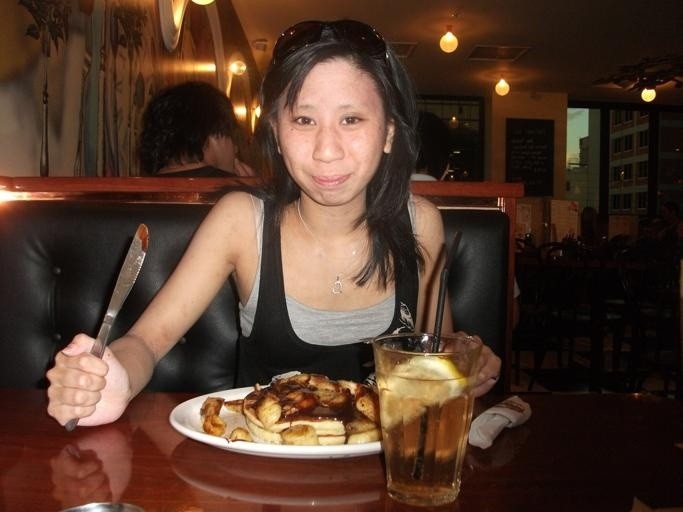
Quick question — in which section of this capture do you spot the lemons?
[410,357,467,407]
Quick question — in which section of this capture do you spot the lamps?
[640,88,656,103]
[438,24,459,54]
[494,74,510,97]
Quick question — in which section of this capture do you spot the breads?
[241,369,383,446]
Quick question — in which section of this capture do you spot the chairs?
[514,238,682,392]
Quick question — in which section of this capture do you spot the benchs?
[0,175,524,389]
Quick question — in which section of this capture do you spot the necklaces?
[297,196,367,296]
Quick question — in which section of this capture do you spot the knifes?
[63,223,149,432]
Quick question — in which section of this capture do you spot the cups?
[370,332,484,507]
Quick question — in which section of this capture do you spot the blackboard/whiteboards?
[508,120,555,199]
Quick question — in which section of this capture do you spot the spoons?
[397,299,427,354]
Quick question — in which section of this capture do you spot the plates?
[168,383,388,461]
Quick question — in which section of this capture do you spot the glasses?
[274,18,392,71]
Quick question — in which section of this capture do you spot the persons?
[45,19,502,429]
[50,391,530,511]
[135,80,257,178]
[565,206,603,260]
[635,199,683,259]
[408,110,450,179]
